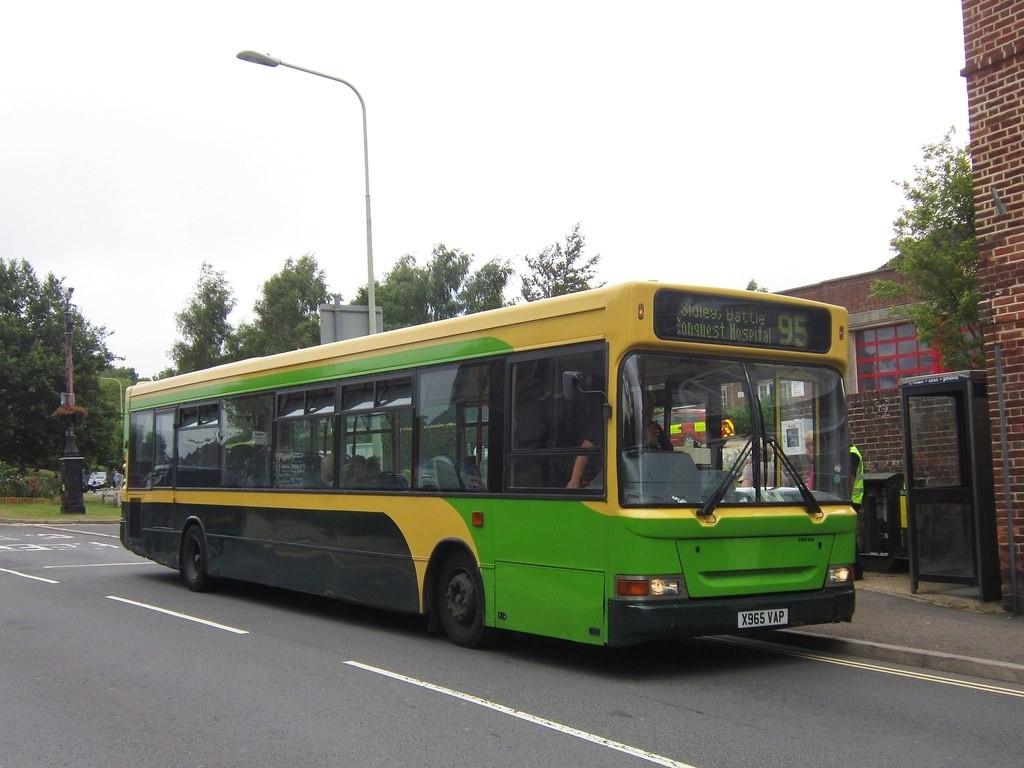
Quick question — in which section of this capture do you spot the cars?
[88,471,110,490]
[82,470,89,492]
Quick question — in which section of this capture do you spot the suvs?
[652,409,734,447]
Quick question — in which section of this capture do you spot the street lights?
[100,378,123,429]
[60,286,86,514]
[236,49,381,470]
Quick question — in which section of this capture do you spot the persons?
[567,385,674,489]
[737,442,792,489]
[299,450,381,489]
[81,463,126,493]
[803,424,866,582]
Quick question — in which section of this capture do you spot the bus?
[119,278,858,650]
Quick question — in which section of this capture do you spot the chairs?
[102,481,126,507]
[141,443,460,491]
